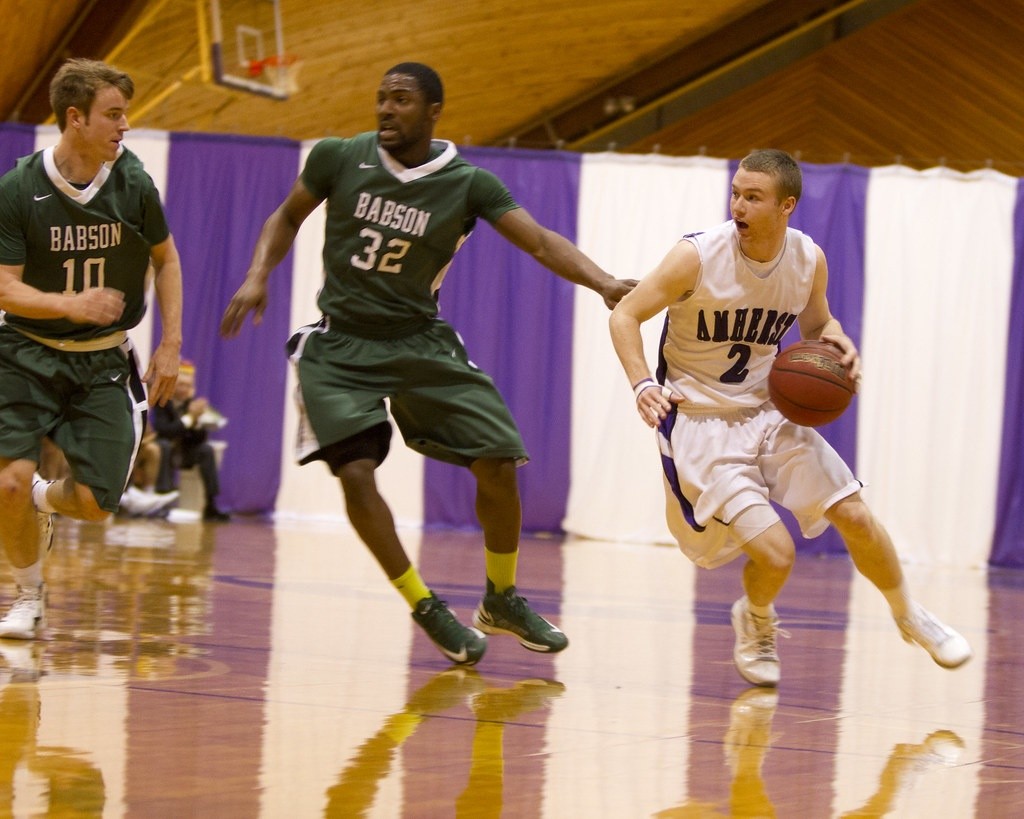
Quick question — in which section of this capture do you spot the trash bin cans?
[177,439,228,512]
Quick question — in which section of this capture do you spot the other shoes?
[201,509,230,521]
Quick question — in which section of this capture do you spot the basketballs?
[766,338,855,428]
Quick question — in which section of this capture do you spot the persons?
[220,62,640,665]
[323,669,565,819]
[43,360,229,521]
[0,638,105,819]
[1,520,211,677]
[659,688,965,819]
[609,151,971,683]
[0,61,183,637]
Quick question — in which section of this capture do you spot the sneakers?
[731,595,791,687]
[143,490,180,515]
[893,604,973,670]
[0,581,47,639]
[31,471,54,563]
[128,498,159,517]
[473,592,568,654]
[407,591,487,666]
